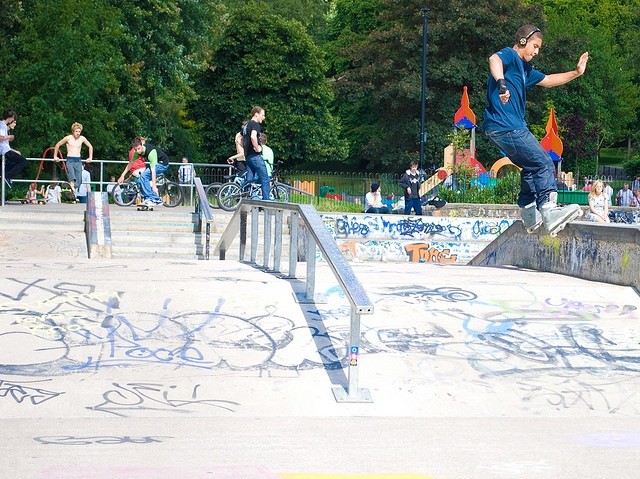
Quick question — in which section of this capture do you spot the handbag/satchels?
[429,196,446,208]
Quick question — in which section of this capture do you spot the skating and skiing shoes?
[536,192,583,237]
[519,200,543,234]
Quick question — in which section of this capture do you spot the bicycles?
[217,178,290,212]
[111,170,184,207]
[203,173,245,210]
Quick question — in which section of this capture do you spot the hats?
[371,182,379,190]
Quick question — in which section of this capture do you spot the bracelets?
[496,79,506,94]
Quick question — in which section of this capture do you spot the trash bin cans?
[320,185,336,197]
[90,181,96,191]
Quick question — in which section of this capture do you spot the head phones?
[519,29,540,46]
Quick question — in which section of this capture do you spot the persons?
[118,138,169,205]
[483,24,589,239]
[632,175,640,194]
[557,169,590,191]
[588,179,610,224]
[54,121,94,202]
[0,108,27,189]
[26,183,45,204]
[617,182,634,207]
[129,137,146,178]
[77,158,91,202]
[364,183,389,214]
[42,177,62,204]
[636,188,640,203]
[243,106,275,201]
[227,133,274,177]
[604,181,613,206]
[177,157,196,206]
[235,119,249,173]
[7,115,17,131]
[107,176,116,194]
[400,161,422,215]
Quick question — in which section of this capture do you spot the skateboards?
[61,182,76,204]
[136,204,154,212]
[17,197,51,205]
